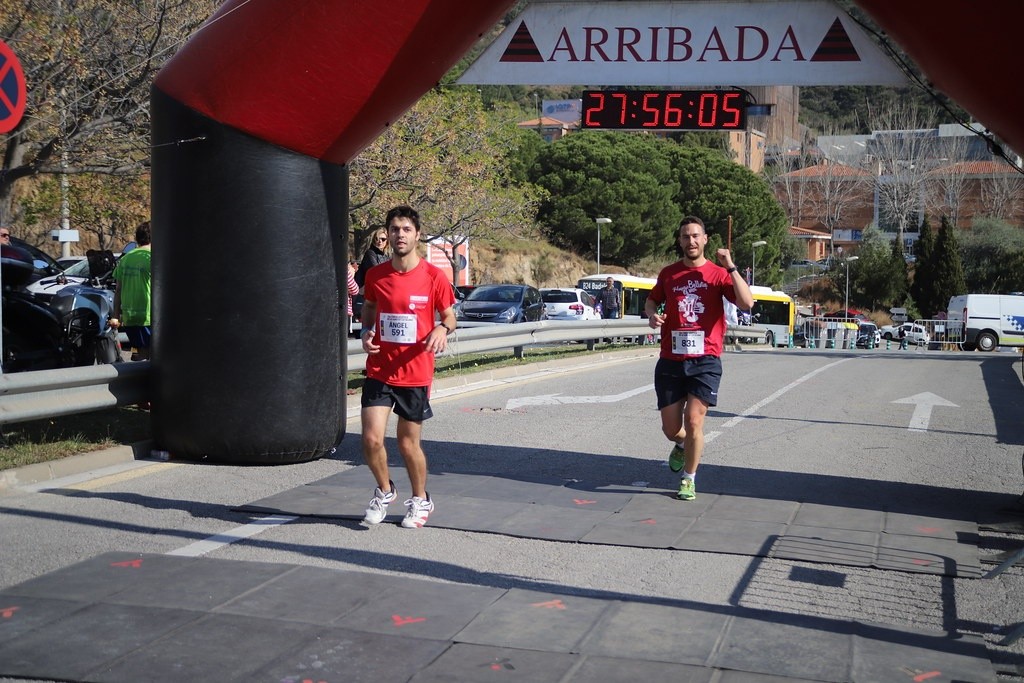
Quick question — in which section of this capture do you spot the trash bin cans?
[802,320,858,349]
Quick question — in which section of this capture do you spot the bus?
[575,274,795,348]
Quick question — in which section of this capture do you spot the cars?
[8,236,124,307]
[450,283,546,325]
[824,309,869,324]
[793,323,809,346]
[778,258,832,272]
[538,288,601,320]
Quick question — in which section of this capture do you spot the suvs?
[879,322,929,346]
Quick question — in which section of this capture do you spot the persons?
[644,216,755,501]
[0,227,10,244]
[109,221,152,360]
[593,277,620,344]
[347,227,390,337]
[898,327,908,350]
[360,206,457,528]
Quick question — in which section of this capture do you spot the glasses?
[375,237,387,241]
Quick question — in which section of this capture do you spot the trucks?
[946,291,1024,352]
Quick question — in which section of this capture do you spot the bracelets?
[440,322,450,334]
[727,267,737,273]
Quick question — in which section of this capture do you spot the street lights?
[752,241,766,285]
[845,256,858,321]
[595,218,613,274]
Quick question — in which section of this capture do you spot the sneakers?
[669,444,686,472]
[676,477,697,500]
[365,480,397,525]
[401,491,435,529]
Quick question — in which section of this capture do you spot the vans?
[857,321,881,348]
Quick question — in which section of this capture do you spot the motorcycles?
[736,308,761,342]
[0,244,137,374]
